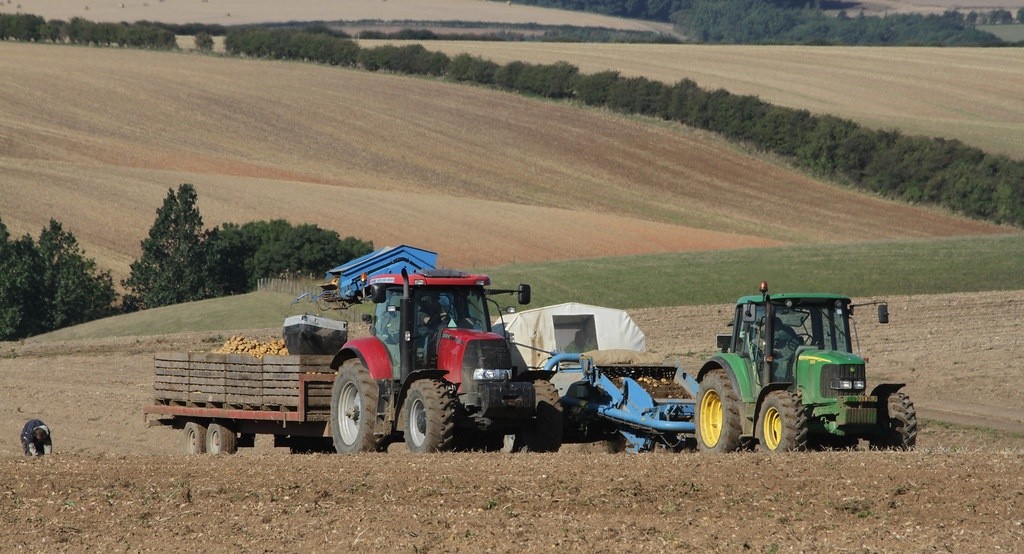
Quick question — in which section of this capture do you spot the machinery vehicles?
[694,282,918,457]
[142,266,565,458]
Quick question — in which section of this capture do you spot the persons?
[417,293,451,347]
[757,317,800,382]
[564,331,594,354]
[21,419,53,456]
